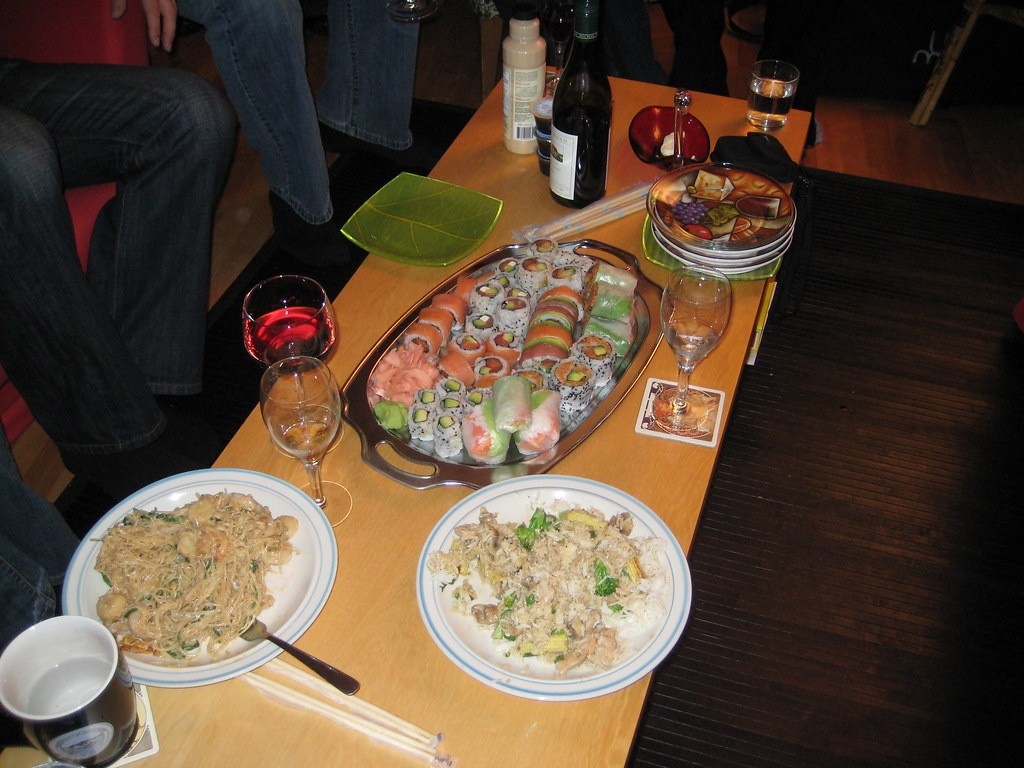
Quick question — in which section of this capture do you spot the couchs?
[0,20,344,514]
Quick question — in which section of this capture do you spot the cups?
[0,615,137,767]
[747,60,799,131]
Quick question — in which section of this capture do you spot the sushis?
[369,234,636,461]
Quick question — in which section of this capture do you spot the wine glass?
[243,275,344,460]
[261,356,353,529]
[546,1,577,96]
[650,265,733,433]
[383,0,439,22]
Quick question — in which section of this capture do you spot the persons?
[175,0,424,226]
[1,0,241,501]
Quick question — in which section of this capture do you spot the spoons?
[241,616,361,695]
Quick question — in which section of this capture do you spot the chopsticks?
[241,671,437,763]
[266,657,441,748]
[539,184,652,236]
[549,200,647,241]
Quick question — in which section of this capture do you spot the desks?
[65,58,813,759]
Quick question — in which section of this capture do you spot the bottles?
[547,1,612,207]
[503,4,547,154]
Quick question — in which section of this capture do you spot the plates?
[61,467,339,689]
[415,474,693,703]
[629,89,710,172]
[339,170,503,268]
[341,239,676,491]
[640,162,797,282]
